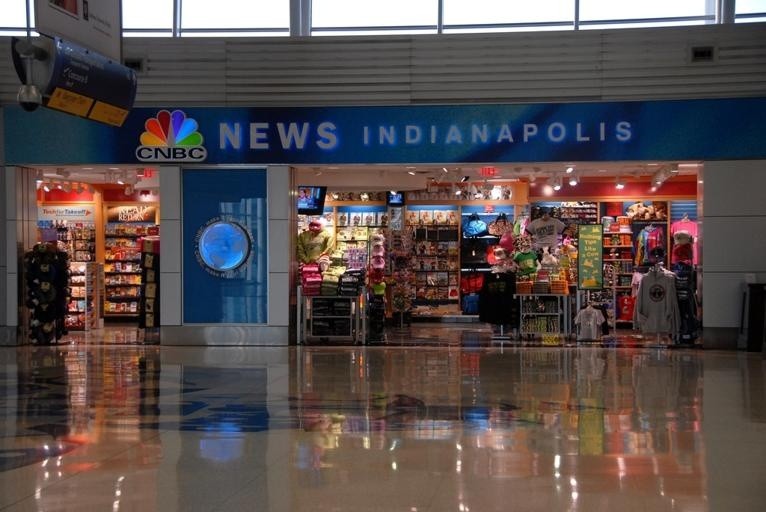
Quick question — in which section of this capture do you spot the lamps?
[397,167,628,198]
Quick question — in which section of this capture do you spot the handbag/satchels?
[487,212,513,236]
[462,214,487,237]
[460,270,484,294]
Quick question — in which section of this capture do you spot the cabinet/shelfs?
[309,294,354,339]
[515,293,562,341]
[337,226,368,266]
[105,233,145,319]
[406,222,459,318]
[67,273,89,331]
[461,212,513,320]
[604,229,635,288]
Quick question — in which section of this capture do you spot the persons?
[526,212,571,260]
[297,188,310,207]
[299,213,388,228]
[442,185,511,200]
[408,210,457,226]
[419,258,426,271]
[430,257,437,272]
[419,240,427,255]
[296,219,334,262]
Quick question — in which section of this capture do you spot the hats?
[308,222,321,232]
[540,207,550,213]
[494,248,505,260]
[370,234,385,295]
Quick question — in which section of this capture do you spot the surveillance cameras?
[16,85,43,112]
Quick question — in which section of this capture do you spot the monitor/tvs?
[386,190,404,207]
[298,186,327,215]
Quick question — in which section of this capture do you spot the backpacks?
[367,297,385,318]
[368,317,385,339]
